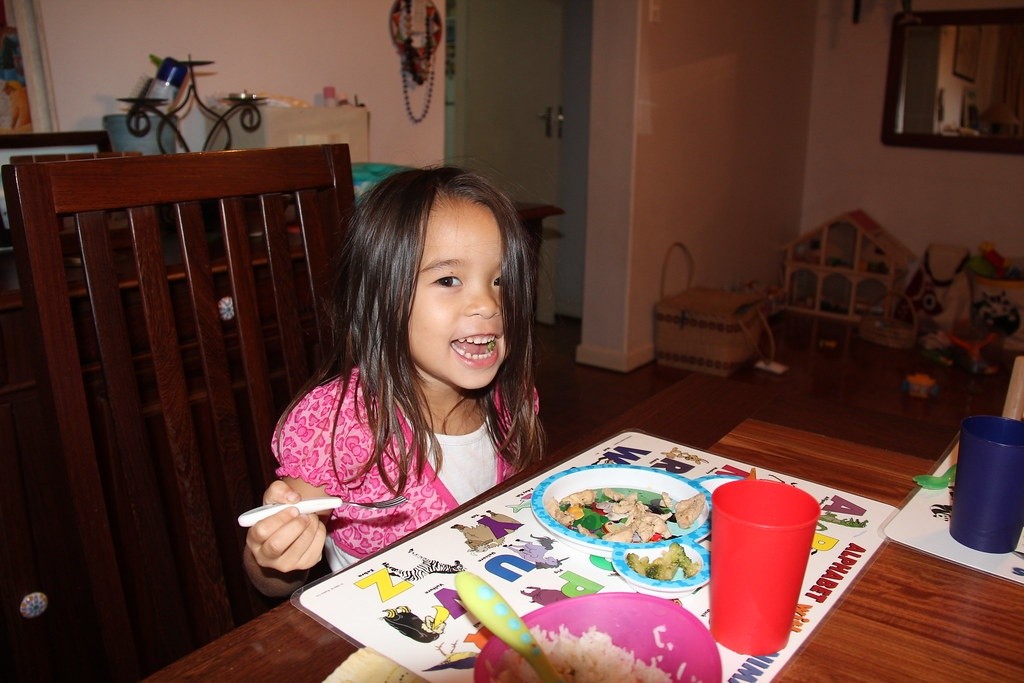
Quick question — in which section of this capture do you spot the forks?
[912,463,957,491]
[237,495,406,527]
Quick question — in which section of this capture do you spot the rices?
[483,624,692,683]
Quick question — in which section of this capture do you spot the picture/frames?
[0,130,111,246]
[954,24,982,83]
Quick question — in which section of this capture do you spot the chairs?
[3,143,359,683]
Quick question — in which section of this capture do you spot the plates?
[611,541,711,599]
[531,464,713,557]
[692,474,746,495]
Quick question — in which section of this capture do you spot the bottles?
[324,86,336,107]
[141,57,187,115]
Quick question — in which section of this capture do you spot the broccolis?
[627,543,698,579]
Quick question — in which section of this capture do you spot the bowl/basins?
[474,591,721,683]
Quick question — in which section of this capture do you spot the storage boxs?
[205,107,370,166]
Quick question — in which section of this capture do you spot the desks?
[142,369,1024,683]
[509,200,555,346]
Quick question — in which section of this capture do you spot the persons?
[242,163,547,597]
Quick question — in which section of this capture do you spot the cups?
[949,414,1024,554]
[709,479,821,655]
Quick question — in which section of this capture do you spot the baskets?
[654,242,775,377]
[859,289,918,348]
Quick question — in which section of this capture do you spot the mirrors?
[880,9,1024,156]
